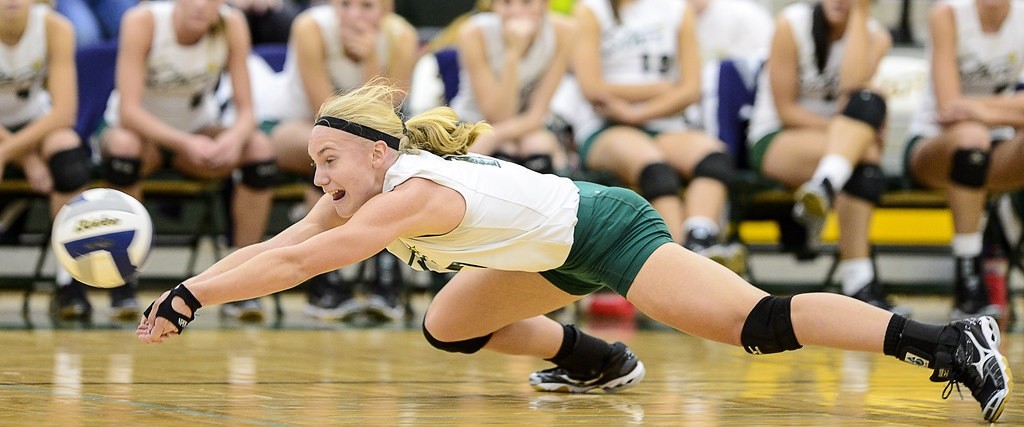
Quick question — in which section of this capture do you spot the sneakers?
[931,316,1013,423]
[683,229,731,265]
[304,278,364,321]
[528,340,646,392]
[791,180,830,228]
[366,283,406,320]
[814,279,914,319]
[946,233,986,320]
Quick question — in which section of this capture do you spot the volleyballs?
[51,187,155,288]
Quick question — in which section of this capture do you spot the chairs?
[0,44,1024,324]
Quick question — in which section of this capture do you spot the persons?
[0,0,1024,331]
[135,75,1013,422]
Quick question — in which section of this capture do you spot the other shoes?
[53,285,92,318]
[223,297,264,323]
[110,284,140,318]
